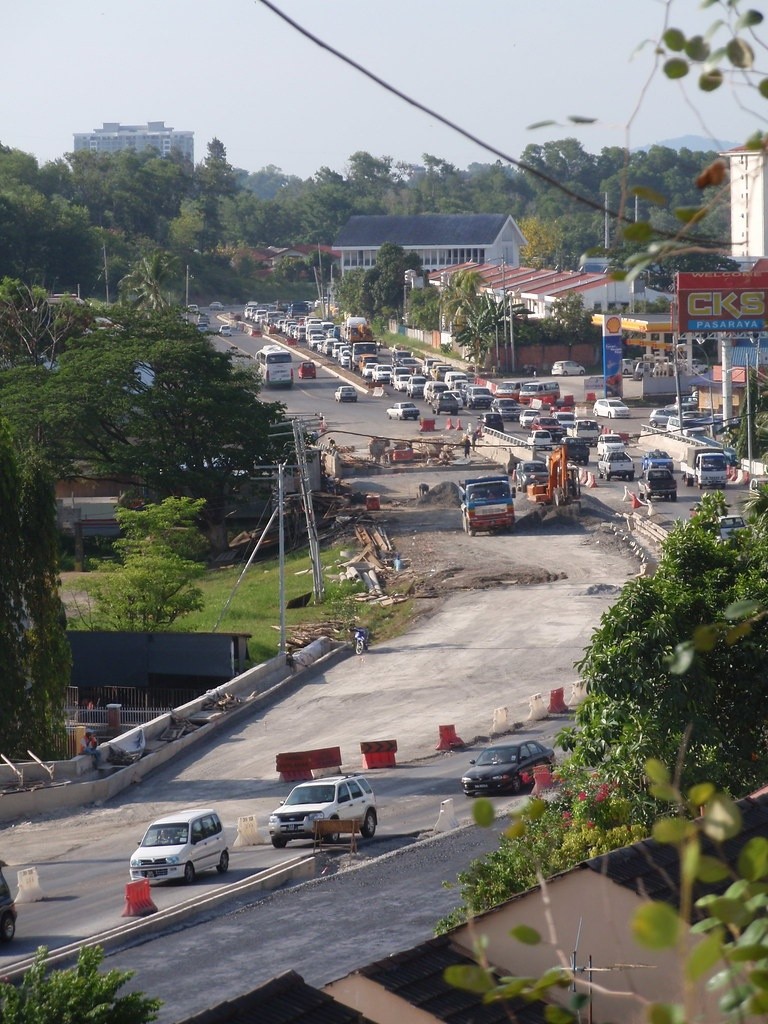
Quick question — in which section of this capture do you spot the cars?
[443,390,466,410]
[648,402,742,437]
[334,385,359,404]
[552,360,585,377]
[0,859,18,945]
[431,393,459,416]
[622,351,710,381]
[592,398,632,419]
[188,302,233,337]
[297,362,316,380]
[460,739,557,797]
[641,449,674,474]
[527,430,553,452]
[128,809,229,886]
[529,416,565,440]
[716,515,746,541]
[243,299,483,405]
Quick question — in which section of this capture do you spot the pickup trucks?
[637,469,678,502]
[556,436,590,464]
[597,451,636,481]
[386,402,420,421]
[566,419,600,447]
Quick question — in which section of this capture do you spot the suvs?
[466,386,495,410]
[490,398,523,421]
[516,460,552,492]
[268,774,377,847]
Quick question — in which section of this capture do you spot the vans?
[551,411,576,429]
[597,434,625,457]
[518,381,561,405]
[494,380,530,400]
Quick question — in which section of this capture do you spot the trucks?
[457,474,517,535]
[680,452,729,489]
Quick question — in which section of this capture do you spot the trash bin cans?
[106,704,122,728]
[367,495,380,510]
[65,726,86,758]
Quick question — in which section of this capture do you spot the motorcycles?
[348,622,371,654]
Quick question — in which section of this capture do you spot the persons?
[80,728,101,769]
[472,430,481,451]
[464,436,471,457]
[418,483,429,498]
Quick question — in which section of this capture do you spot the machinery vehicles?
[527,445,582,507]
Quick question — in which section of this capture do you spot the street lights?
[488,254,509,374]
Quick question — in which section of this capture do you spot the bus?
[254,345,294,389]
